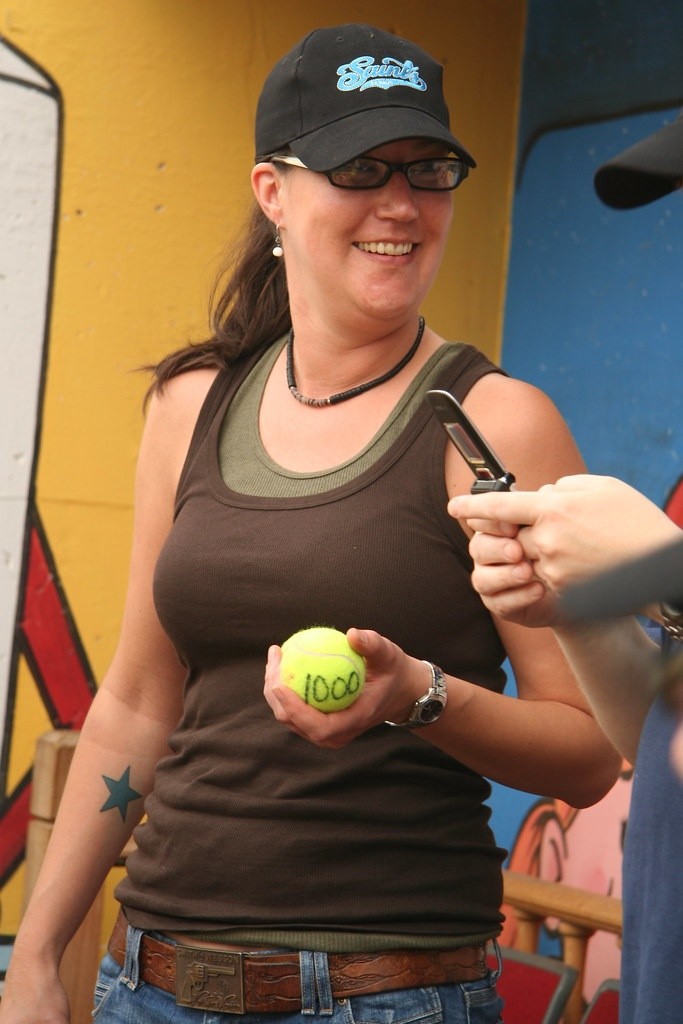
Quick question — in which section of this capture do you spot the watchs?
[384,658,448,730]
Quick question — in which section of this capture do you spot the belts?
[107,905,488,1014]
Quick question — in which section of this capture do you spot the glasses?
[269,155,469,191]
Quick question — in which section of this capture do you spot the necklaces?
[286,316,426,408]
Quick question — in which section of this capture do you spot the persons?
[0,18,623,1024]
[447,470,683,1024]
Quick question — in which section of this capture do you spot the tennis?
[279,623,366,711]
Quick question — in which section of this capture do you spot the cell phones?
[426,388,519,494]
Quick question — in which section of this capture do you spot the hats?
[593,115,681,210]
[255,24,478,173]
